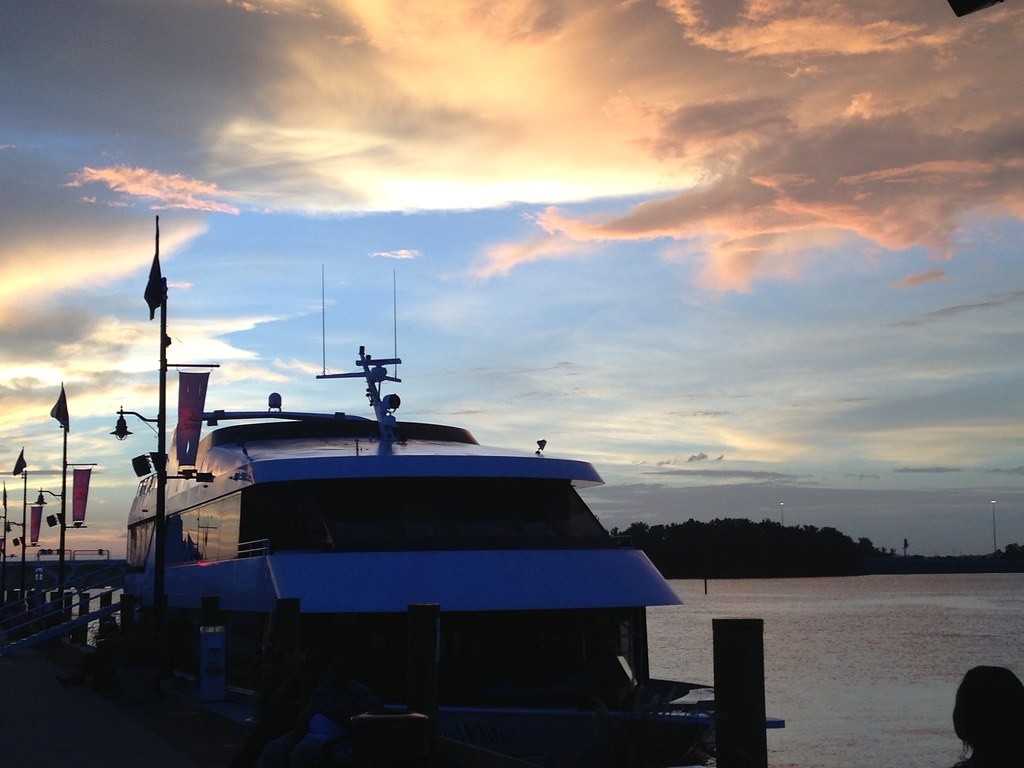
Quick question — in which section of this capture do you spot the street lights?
[3,517,28,606]
[990,500,998,557]
[110,410,172,611]
[778,502,786,525]
[36,487,66,604]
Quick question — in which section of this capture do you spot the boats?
[121,266,788,768]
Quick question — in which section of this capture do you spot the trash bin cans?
[199,626,228,702]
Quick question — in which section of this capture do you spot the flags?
[30,506,43,542]
[176,373,210,467]
[51,387,70,433]
[145,222,164,320]
[73,470,91,521]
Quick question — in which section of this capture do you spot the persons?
[255,658,385,768]
[952,664,1024,768]
